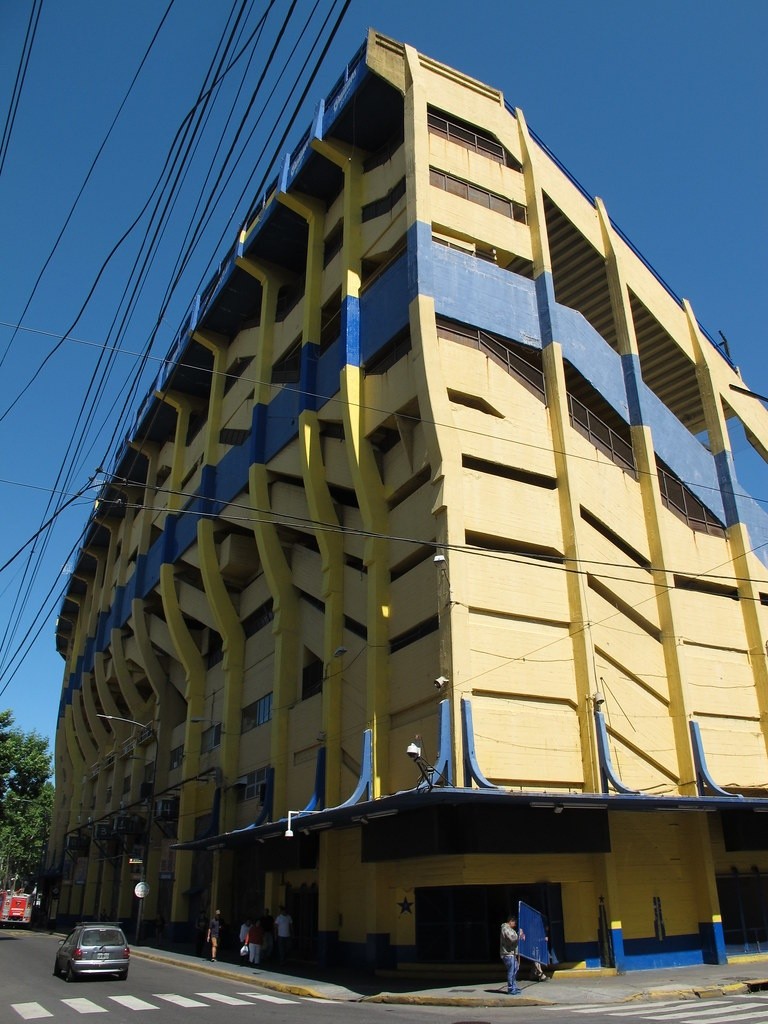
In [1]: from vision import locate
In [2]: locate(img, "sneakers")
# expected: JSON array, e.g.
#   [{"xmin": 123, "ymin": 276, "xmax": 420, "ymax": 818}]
[
  {"xmin": 514, "ymin": 988, "xmax": 521, "ymax": 993},
  {"xmin": 508, "ymin": 990, "xmax": 517, "ymax": 995}
]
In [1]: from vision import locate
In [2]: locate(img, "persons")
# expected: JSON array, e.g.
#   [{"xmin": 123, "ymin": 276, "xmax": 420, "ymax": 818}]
[
  {"xmin": 154, "ymin": 911, "xmax": 165, "ymax": 946},
  {"xmin": 502, "ymin": 916, "xmax": 524, "ymax": 995},
  {"xmin": 101, "ymin": 907, "xmax": 116, "ymax": 921},
  {"xmin": 196, "ymin": 911, "xmax": 209, "ymax": 940},
  {"xmin": 207, "ymin": 910, "xmax": 224, "ymax": 962},
  {"xmin": 240, "ymin": 907, "xmax": 292, "ymax": 965}
]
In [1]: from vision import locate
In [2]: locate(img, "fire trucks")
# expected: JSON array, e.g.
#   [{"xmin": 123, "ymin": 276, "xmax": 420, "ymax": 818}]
[{"xmin": 0, "ymin": 889, "xmax": 34, "ymax": 930}]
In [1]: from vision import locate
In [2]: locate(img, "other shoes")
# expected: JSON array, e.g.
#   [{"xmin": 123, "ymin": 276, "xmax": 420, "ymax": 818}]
[{"xmin": 211, "ymin": 959, "xmax": 218, "ymax": 962}]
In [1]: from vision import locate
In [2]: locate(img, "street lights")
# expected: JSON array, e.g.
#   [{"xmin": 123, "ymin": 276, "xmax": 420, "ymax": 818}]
[
  {"xmin": 13, "ymin": 797, "xmax": 47, "ymax": 880},
  {"xmin": 96, "ymin": 714, "xmax": 159, "ymax": 945}
]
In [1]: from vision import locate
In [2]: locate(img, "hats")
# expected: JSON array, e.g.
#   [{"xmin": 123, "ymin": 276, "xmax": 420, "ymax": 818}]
[{"xmin": 216, "ymin": 909, "xmax": 222, "ymax": 914}]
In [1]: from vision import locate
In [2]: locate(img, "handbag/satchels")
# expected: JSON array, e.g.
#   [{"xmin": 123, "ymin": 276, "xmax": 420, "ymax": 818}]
[{"xmin": 240, "ymin": 945, "xmax": 249, "ymax": 956}]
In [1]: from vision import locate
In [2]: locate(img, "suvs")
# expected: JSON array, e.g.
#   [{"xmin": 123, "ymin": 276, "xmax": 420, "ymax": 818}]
[{"xmin": 54, "ymin": 922, "xmax": 130, "ymax": 981}]
